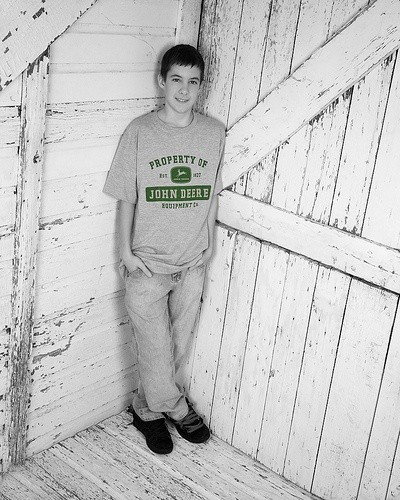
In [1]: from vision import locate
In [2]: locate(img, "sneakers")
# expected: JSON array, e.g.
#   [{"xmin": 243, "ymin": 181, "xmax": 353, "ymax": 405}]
[
  {"xmin": 161, "ymin": 397, "xmax": 210, "ymax": 443},
  {"xmin": 132, "ymin": 409, "xmax": 173, "ymax": 454}
]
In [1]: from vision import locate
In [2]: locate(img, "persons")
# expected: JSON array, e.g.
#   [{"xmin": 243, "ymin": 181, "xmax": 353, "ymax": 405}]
[{"xmin": 103, "ymin": 43, "xmax": 226, "ymax": 455}]
